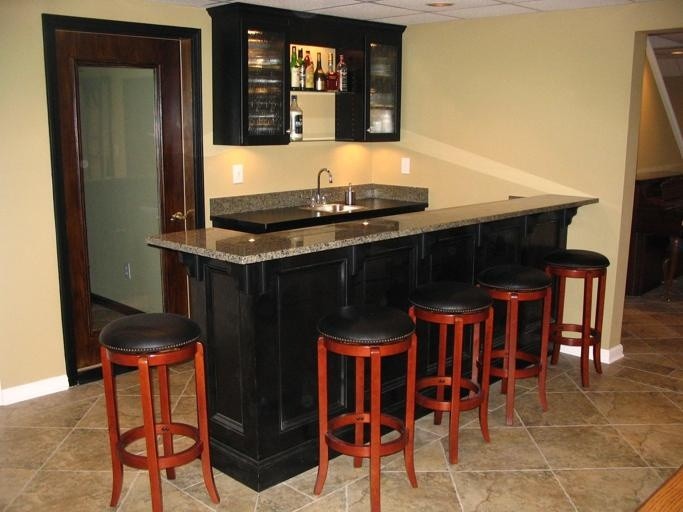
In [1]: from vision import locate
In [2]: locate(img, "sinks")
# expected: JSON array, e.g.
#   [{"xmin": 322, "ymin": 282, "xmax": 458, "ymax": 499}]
[{"xmin": 302, "ymin": 203, "xmax": 364, "ymax": 213}]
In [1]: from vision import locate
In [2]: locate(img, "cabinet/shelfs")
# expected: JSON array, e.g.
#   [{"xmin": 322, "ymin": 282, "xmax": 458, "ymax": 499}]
[
  {"xmin": 206, "ymin": 3, "xmax": 290, "ymax": 145},
  {"xmin": 290, "ymin": 87, "xmax": 354, "ymax": 142},
  {"xmin": 334, "ymin": 17, "xmax": 407, "ymax": 142}
]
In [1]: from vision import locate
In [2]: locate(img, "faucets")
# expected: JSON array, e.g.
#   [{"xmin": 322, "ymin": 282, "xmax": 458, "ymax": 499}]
[{"xmin": 318, "ymin": 169, "xmax": 333, "ymax": 195}]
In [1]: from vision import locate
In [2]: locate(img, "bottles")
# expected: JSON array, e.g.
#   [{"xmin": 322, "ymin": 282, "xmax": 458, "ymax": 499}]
[
  {"xmin": 290, "ymin": 45, "xmax": 348, "ymax": 91},
  {"xmin": 289, "ymin": 96, "xmax": 303, "ymax": 142},
  {"xmin": 344, "ymin": 183, "xmax": 355, "ymax": 205}
]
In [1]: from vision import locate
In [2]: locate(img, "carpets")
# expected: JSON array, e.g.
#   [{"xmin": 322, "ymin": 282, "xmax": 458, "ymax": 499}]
[{"xmin": 635, "ymin": 465, "xmax": 683, "ymax": 512}]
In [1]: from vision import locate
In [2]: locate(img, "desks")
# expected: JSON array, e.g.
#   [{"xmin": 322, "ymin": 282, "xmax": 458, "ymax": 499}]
[{"xmin": 625, "ymin": 168, "xmax": 683, "ymax": 296}]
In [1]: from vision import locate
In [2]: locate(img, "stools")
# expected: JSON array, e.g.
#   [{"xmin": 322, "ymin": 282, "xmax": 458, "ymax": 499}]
[
  {"xmin": 664, "ymin": 234, "xmax": 683, "ymax": 302},
  {"xmin": 469, "ymin": 264, "xmax": 552, "ymax": 426},
  {"xmin": 313, "ymin": 305, "xmax": 418, "ymax": 512},
  {"xmin": 409, "ymin": 280, "xmax": 494, "ymax": 465},
  {"xmin": 544, "ymin": 250, "xmax": 610, "ymax": 387},
  {"xmin": 98, "ymin": 313, "xmax": 220, "ymax": 512}
]
